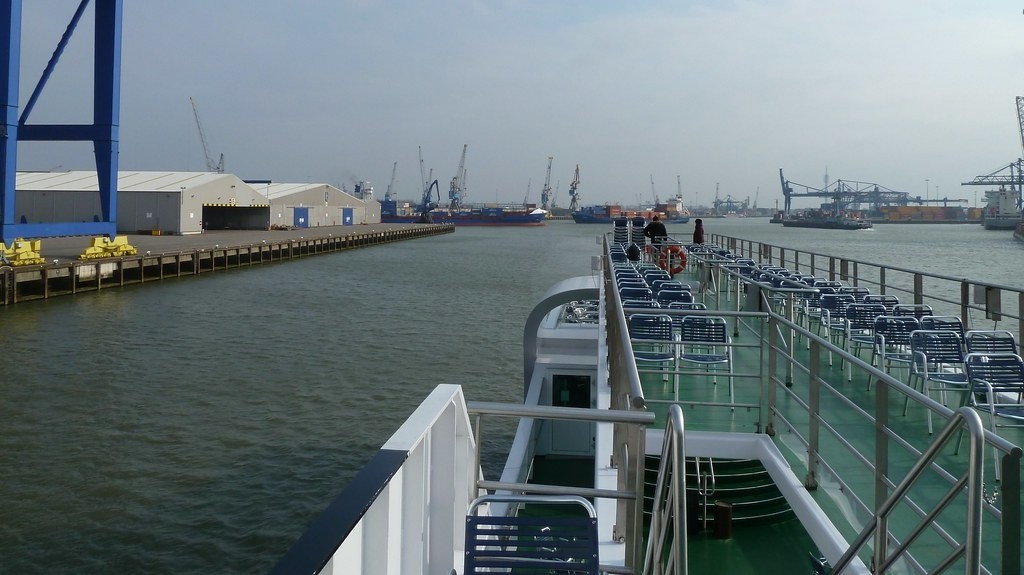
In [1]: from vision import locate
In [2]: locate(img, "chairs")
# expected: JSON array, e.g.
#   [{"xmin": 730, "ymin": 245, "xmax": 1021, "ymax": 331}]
[
  {"xmin": 462, "ymin": 493, "xmax": 600, "ymax": 575},
  {"xmin": 608, "ymin": 216, "xmax": 1023, "ymax": 484}
]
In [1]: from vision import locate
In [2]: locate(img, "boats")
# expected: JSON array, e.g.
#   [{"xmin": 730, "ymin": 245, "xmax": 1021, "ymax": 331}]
[
  {"xmin": 768, "ymin": 214, "xmax": 783, "ymax": 223},
  {"xmin": 783, "ymin": 208, "xmax": 873, "ymax": 230},
  {"xmin": 979, "ymin": 185, "xmax": 1023, "ymax": 230},
  {"xmin": 572, "ymin": 195, "xmax": 692, "ymax": 226},
  {"xmin": 381, "ymin": 202, "xmax": 549, "ymax": 229}
]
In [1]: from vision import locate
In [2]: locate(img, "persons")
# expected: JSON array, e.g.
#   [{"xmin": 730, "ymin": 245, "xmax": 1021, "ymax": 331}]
[
  {"xmin": 693, "ymin": 219, "xmax": 704, "ymax": 266},
  {"xmin": 644, "ymin": 215, "xmax": 667, "ymax": 263}
]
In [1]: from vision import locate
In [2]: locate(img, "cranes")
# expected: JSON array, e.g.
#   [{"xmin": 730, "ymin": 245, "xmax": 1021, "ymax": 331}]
[
  {"xmin": 537, "ymin": 155, "xmax": 563, "ymax": 219},
  {"xmin": 714, "ymin": 182, "xmax": 751, "ymax": 217},
  {"xmin": 773, "ymin": 169, "xmax": 970, "ymax": 216},
  {"xmin": 569, "ymin": 165, "xmax": 583, "ymax": 214},
  {"xmin": 377, "ymin": 143, "xmax": 474, "ymax": 217},
  {"xmin": 958, "ymin": 96, "xmax": 1024, "ymax": 212},
  {"xmin": 189, "ymin": 96, "xmax": 225, "ymax": 174}
]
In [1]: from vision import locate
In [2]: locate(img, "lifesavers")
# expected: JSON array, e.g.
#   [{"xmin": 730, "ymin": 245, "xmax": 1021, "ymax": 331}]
[{"xmin": 659, "ymin": 248, "xmax": 686, "ymax": 273}]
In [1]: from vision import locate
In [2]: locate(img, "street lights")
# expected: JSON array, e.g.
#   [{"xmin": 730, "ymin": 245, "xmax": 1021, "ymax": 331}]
[{"xmin": 926, "ymin": 179, "xmax": 930, "ymax": 207}]
